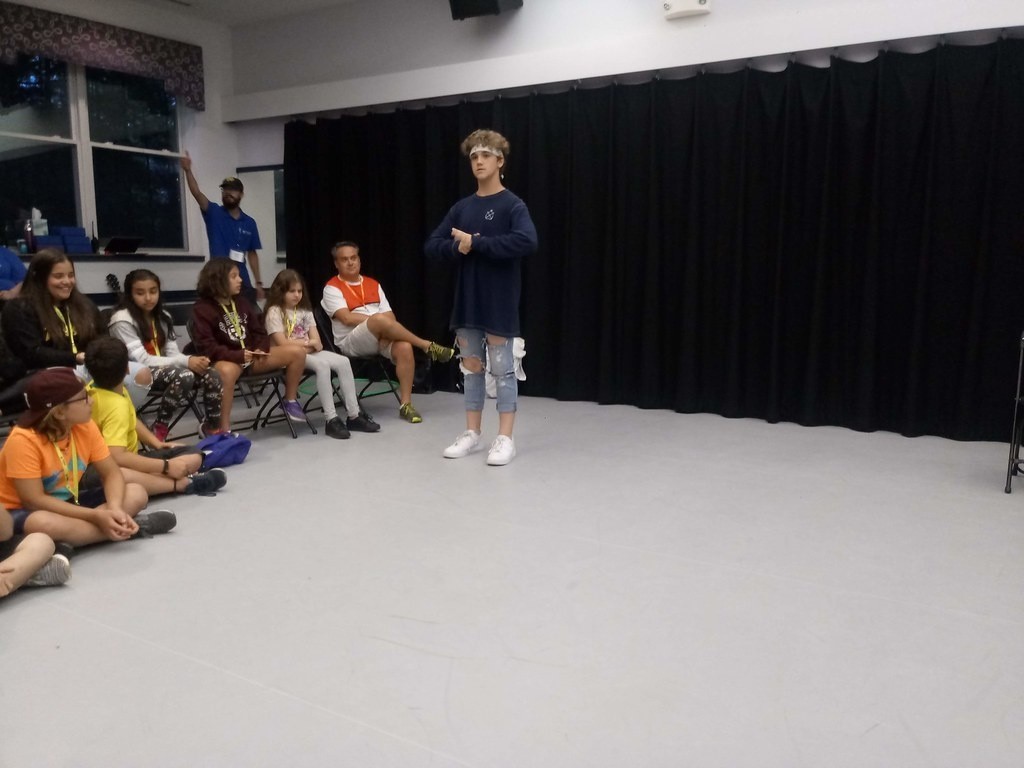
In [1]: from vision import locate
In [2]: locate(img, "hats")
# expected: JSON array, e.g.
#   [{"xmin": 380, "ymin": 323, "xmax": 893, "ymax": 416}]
[
  {"xmin": 16, "ymin": 366, "xmax": 86, "ymax": 428},
  {"xmin": 219, "ymin": 178, "xmax": 244, "ymax": 192}
]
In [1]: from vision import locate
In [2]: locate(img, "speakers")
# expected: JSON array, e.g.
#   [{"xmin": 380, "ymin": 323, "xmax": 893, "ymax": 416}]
[{"xmin": 449, "ymin": 0, "xmax": 523, "ymax": 21}]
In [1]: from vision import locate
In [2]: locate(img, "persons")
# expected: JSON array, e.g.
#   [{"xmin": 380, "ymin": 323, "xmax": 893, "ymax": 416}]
[
  {"xmin": 107, "ymin": 270, "xmax": 224, "ymax": 442},
  {"xmin": 0, "ymin": 241, "xmax": 28, "ymax": 300},
  {"xmin": 320, "ymin": 242, "xmax": 456, "ymax": 423},
  {"xmin": 1, "ymin": 503, "xmax": 70, "ymax": 599},
  {"xmin": 188, "ymin": 255, "xmax": 306, "ymax": 433},
  {"xmin": 22, "ymin": 248, "xmax": 112, "ymax": 367},
  {"xmin": 422, "ymin": 130, "xmax": 537, "ymax": 466},
  {"xmin": 179, "ymin": 149, "xmax": 265, "ymax": 302},
  {"xmin": 81, "ymin": 336, "xmax": 227, "ymax": 497},
  {"xmin": 0, "ymin": 367, "xmax": 176, "ymax": 546},
  {"xmin": 263, "ymin": 268, "xmax": 380, "ymax": 439}
]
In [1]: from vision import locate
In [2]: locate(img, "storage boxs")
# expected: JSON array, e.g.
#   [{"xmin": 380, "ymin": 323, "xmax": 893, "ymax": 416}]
[
  {"xmin": 36, "ymin": 245, "xmax": 65, "ymax": 253},
  {"xmin": 51, "ymin": 227, "xmax": 86, "ymax": 237},
  {"xmin": 63, "ymin": 237, "xmax": 90, "ymax": 245},
  {"xmin": 33, "ymin": 236, "xmax": 63, "ymax": 245},
  {"xmin": 66, "ymin": 245, "xmax": 92, "ymax": 253}
]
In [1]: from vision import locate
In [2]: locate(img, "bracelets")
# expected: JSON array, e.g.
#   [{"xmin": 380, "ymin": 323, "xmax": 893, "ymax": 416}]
[
  {"xmin": 163, "ymin": 457, "xmax": 168, "ymax": 475},
  {"xmin": 256, "ymin": 281, "xmax": 262, "ymax": 285}
]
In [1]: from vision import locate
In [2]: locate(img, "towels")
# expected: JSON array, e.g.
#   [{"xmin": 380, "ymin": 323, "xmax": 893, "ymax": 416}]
[{"xmin": 484, "ymin": 336, "xmax": 527, "ymax": 399}]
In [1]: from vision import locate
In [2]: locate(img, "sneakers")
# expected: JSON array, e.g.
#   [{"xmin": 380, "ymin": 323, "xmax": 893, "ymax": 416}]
[
  {"xmin": 346, "ymin": 411, "xmax": 380, "ymax": 432},
  {"xmin": 487, "ymin": 434, "xmax": 515, "ymax": 465},
  {"xmin": 443, "ymin": 430, "xmax": 484, "ymax": 459},
  {"xmin": 425, "ymin": 343, "xmax": 455, "ymax": 362},
  {"xmin": 129, "ymin": 509, "xmax": 177, "ymax": 539},
  {"xmin": 55, "ymin": 543, "xmax": 75, "ymax": 560},
  {"xmin": 151, "ymin": 421, "xmax": 168, "ymax": 442},
  {"xmin": 399, "ymin": 402, "xmax": 422, "ymax": 423},
  {"xmin": 280, "ymin": 400, "xmax": 307, "ymax": 421},
  {"xmin": 201, "ymin": 423, "xmax": 220, "ymax": 437},
  {"xmin": 325, "ymin": 416, "xmax": 351, "ymax": 439},
  {"xmin": 185, "ymin": 467, "xmax": 228, "ymax": 497},
  {"xmin": 19, "ymin": 555, "xmax": 71, "ymax": 587}
]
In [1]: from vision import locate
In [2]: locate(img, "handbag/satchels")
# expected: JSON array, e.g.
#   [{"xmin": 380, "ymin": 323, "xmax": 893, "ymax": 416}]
[{"xmin": 195, "ymin": 430, "xmax": 251, "ymax": 472}]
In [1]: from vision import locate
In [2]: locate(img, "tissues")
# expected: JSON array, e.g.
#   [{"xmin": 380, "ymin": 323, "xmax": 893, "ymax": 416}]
[{"xmin": 30, "ymin": 207, "xmax": 49, "ymax": 237}]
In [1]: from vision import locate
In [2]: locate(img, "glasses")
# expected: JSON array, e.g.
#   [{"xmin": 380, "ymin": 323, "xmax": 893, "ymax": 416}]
[{"xmin": 64, "ymin": 390, "xmax": 91, "ymax": 406}]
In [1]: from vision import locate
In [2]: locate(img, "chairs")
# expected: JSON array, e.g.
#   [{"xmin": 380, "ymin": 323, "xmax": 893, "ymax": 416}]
[{"xmin": 80, "ymin": 287, "xmax": 401, "ymax": 453}]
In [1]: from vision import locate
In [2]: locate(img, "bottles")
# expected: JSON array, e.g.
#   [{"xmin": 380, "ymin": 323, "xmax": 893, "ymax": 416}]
[
  {"xmin": 19, "ymin": 239, "xmax": 27, "ymax": 254},
  {"xmin": 24, "ymin": 219, "xmax": 33, "ymax": 250}
]
